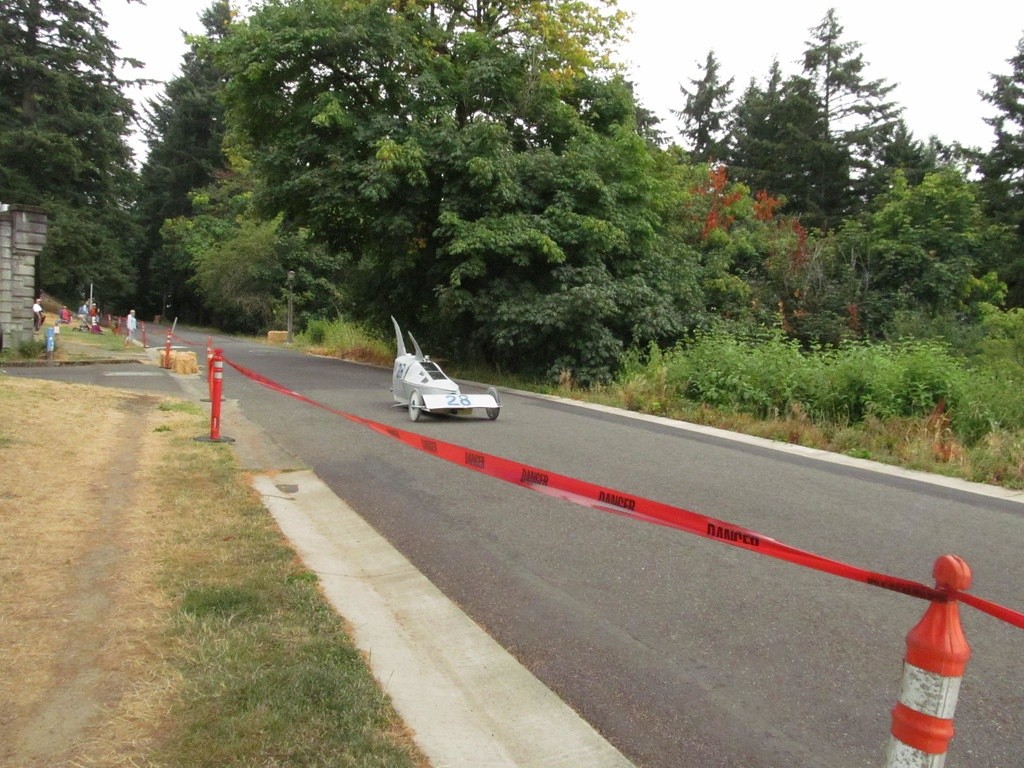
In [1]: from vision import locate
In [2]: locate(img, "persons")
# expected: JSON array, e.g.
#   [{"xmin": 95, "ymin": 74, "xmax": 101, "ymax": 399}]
[
  {"xmin": 123, "ymin": 309, "xmax": 137, "ymax": 346},
  {"xmin": 77, "ymin": 300, "xmax": 105, "ymax": 335},
  {"xmin": 59, "ymin": 305, "xmax": 73, "ymax": 324},
  {"xmin": 33, "ymin": 298, "xmax": 43, "ymax": 335}
]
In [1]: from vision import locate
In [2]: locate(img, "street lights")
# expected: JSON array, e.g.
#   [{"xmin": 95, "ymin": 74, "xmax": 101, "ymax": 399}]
[{"xmin": 285, "ymin": 271, "xmax": 297, "ymax": 347}]
